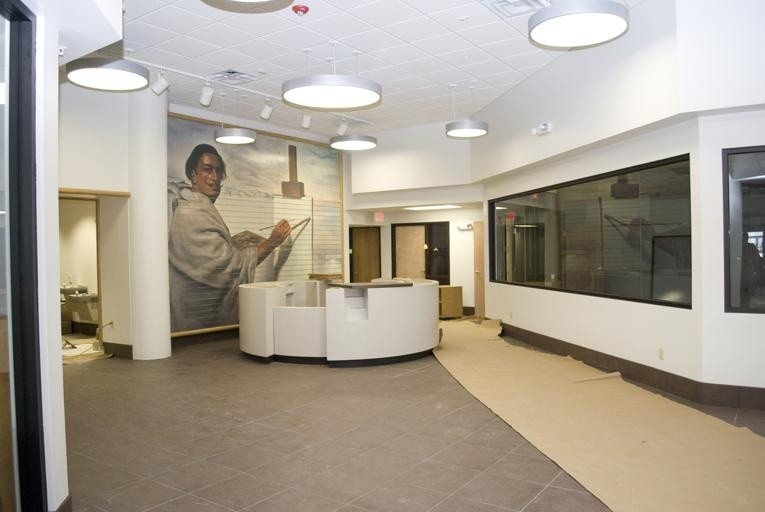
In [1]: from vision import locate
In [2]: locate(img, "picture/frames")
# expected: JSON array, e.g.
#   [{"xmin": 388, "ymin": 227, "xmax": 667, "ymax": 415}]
[{"xmin": 168, "ymin": 111, "xmax": 345, "ymax": 339}]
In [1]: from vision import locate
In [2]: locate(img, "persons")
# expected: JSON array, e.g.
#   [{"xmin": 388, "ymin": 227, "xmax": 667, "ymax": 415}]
[{"xmin": 168, "ymin": 143, "xmax": 291, "ymax": 333}]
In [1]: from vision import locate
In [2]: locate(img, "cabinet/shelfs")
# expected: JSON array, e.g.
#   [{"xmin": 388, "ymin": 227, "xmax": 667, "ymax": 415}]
[{"xmin": 438, "ymin": 284, "xmax": 463, "ymax": 320}]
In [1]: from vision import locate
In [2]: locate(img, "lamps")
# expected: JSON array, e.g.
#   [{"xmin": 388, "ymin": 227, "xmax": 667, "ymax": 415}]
[
  {"xmin": 259, "ymin": 98, "xmax": 273, "ymax": 121},
  {"xmin": 65, "ymin": 46, "xmax": 149, "ymax": 92},
  {"xmin": 446, "ymin": 85, "xmax": 488, "ymax": 140},
  {"xmin": 337, "ymin": 118, "xmax": 347, "ymax": 136},
  {"xmin": 301, "ymin": 112, "xmax": 312, "ymax": 129},
  {"xmin": 526, "ymin": 1, "xmax": 630, "ymax": 52},
  {"xmin": 330, "ymin": 112, "xmax": 378, "ymax": 152},
  {"xmin": 199, "ymin": 81, "xmax": 214, "ymax": 107},
  {"xmin": 150, "ymin": 69, "xmax": 170, "ymax": 96},
  {"xmin": 281, "ymin": 42, "xmax": 381, "ymax": 110},
  {"xmin": 215, "ymin": 93, "xmax": 256, "ymax": 145}
]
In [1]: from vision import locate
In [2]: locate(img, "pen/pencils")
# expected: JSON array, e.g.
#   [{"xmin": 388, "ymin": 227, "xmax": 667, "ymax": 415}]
[{"xmin": 260, "ymin": 219, "xmax": 296, "ymax": 231}]
